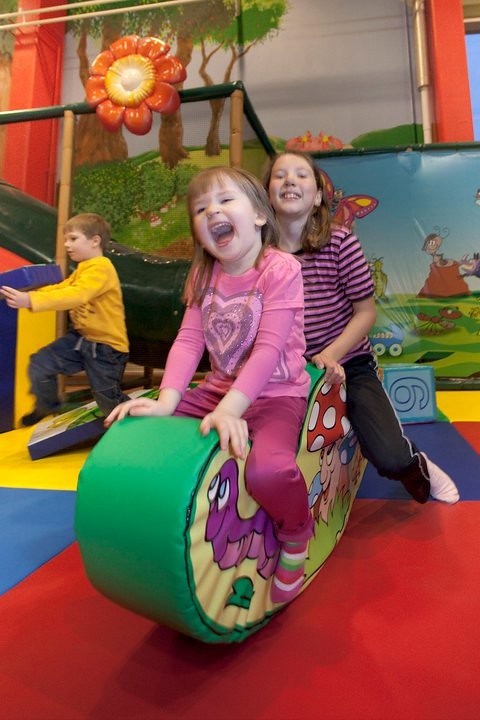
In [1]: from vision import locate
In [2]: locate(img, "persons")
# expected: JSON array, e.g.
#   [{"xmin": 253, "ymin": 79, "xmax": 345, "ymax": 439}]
[
  {"xmin": 104, "ymin": 165, "xmax": 312, "ymax": 602},
  {"xmin": 0, "ymin": 213, "xmax": 133, "ymax": 426},
  {"xmin": 261, "ymin": 148, "xmax": 459, "ymax": 504}
]
